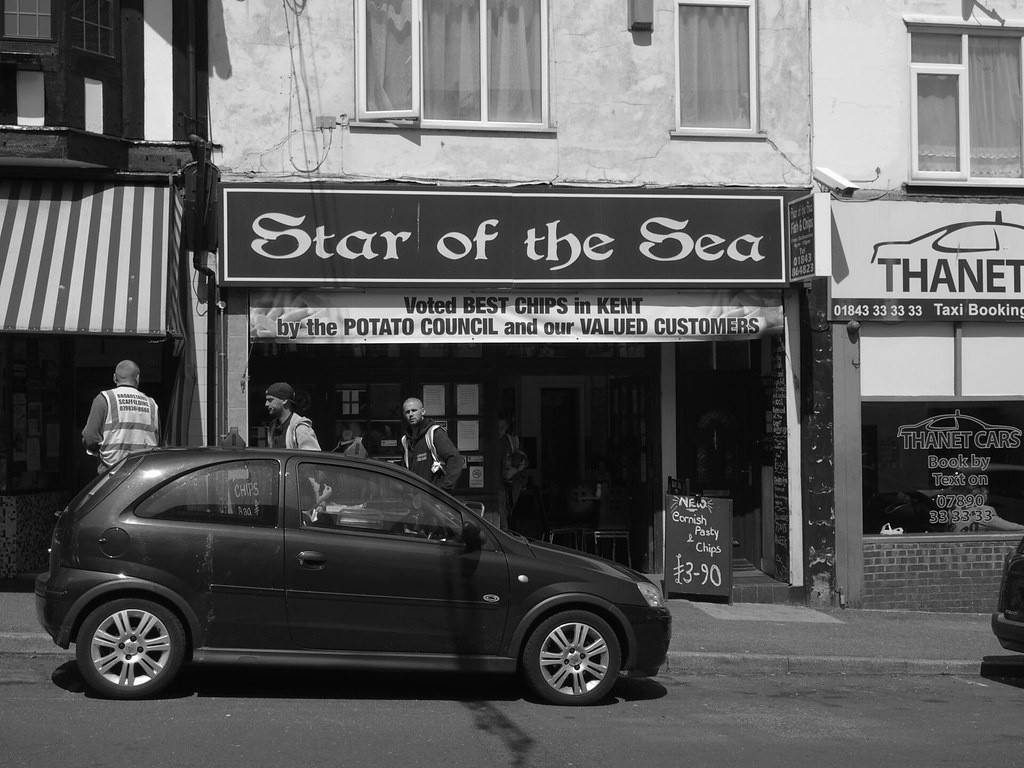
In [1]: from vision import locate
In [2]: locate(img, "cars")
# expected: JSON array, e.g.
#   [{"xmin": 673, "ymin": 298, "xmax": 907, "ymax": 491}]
[
  {"xmin": 917, "ymin": 463, "xmax": 1024, "ymax": 509},
  {"xmin": 991, "ymin": 534, "xmax": 1024, "ymax": 650},
  {"xmin": 35, "ymin": 445, "xmax": 673, "ymax": 706}
]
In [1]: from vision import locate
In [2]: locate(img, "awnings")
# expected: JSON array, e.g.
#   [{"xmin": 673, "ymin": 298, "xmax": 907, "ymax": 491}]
[{"xmin": 0, "ymin": 173, "xmax": 187, "ymax": 354}]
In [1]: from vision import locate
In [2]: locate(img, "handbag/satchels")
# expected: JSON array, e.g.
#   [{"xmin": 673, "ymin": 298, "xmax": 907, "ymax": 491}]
[{"xmin": 291, "ymin": 422, "xmax": 332, "ymax": 511}]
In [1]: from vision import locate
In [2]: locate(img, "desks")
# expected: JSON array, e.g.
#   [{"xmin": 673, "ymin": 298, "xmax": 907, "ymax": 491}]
[{"xmin": 559, "ymin": 494, "xmax": 630, "ymax": 556}]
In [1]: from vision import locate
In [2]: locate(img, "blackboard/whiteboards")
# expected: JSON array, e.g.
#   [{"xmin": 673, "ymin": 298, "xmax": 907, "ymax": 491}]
[{"xmin": 662, "ymin": 494, "xmax": 734, "ymax": 598}]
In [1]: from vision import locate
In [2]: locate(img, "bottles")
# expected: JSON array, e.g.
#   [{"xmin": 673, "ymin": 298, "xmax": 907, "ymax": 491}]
[{"xmin": 596, "ymin": 483, "xmax": 601, "ymax": 496}]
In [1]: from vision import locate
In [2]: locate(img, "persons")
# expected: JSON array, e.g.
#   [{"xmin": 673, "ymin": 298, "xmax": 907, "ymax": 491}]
[
  {"xmin": 946, "ymin": 482, "xmax": 1024, "ymax": 531},
  {"xmin": 265, "ymin": 383, "xmax": 332, "ymax": 513},
  {"xmin": 498, "ymin": 413, "xmax": 536, "ymax": 532},
  {"xmin": 81, "ymin": 361, "xmax": 162, "ymax": 476},
  {"xmin": 399, "ymin": 398, "xmax": 461, "ymax": 497}
]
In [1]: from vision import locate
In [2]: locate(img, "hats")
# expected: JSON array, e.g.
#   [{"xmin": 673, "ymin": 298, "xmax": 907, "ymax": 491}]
[{"xmin": 264, "ymin": 382, "xmax": 298, "ymax": 406}]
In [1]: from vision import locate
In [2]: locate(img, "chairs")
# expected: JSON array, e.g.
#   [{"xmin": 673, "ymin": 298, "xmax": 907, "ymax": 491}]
[
  {"xmin": 261, "ymin": 476, "xmax": 317, "ymax": 528},
  {"xmin": 532, "ymin": 486, "xmax": 632, "ymax": 570}
]
